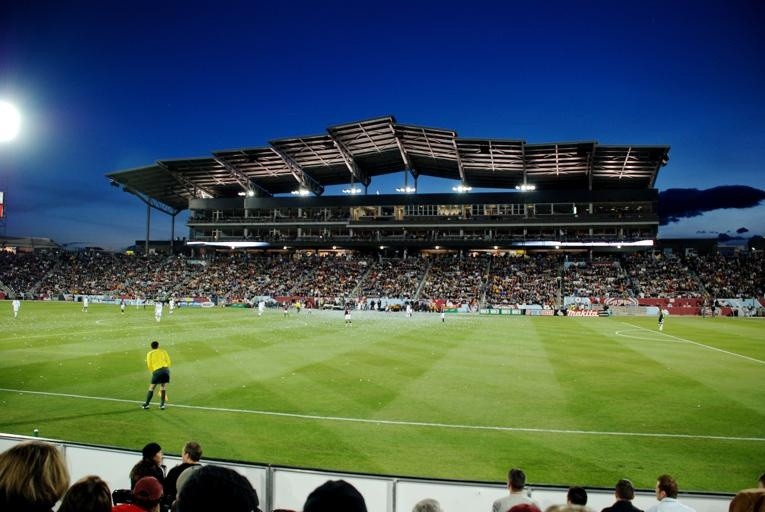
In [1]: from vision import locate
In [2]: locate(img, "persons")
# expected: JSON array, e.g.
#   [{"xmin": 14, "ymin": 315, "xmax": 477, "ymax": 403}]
[
  {"xmin": 729, "ymin": 472, "xmax": 764, "ymax": 512},
  {"xmin": 492, "ymin": 468, "xmax": 596, "ymax": 512},
  {"xmin": 601, "ymin": 479, "xmax": 645, "ymax": 512},
  {"xmin": 1, "ymin": 441, "xmax": 368, "ymax": 512},
  {"xmin": 412, "ymin": 498, "xmax": 444, "ymax": 512},
  {"xmin": 645, "ymin": 474, "xmax": 697, "ymax": 512},
  {"xmin": 141, "ymin": 340, "xmax": 172, "ymax": 410},
  {"xmin": 1, "ymin": 249, "xmax": 765, "ymax": 331}
]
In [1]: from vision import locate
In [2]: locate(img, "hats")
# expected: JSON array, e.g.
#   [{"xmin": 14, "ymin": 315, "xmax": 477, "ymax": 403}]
[{"xmin": 134, "ymin": 476, "xmax": 162, "ymax": 500}]
